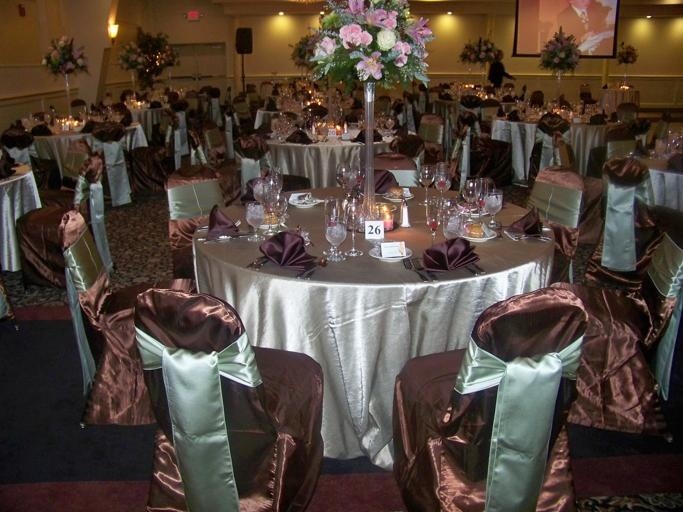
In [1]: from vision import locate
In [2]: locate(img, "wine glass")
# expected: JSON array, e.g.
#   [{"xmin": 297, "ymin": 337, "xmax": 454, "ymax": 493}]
[
  {"xmin": 419, "ymin": 163, "xmax": 503, "ymax": 242},
  {"xmin": 245, "ymin": 167, "xmax": 288, "ymax": 242},
  {"xmin": 655, "ymin": 139, "xmax": 666, "ymax": 162},
  {"xmin": 323, "ymin": 163, "xmax": 364, "ymax": 262}
]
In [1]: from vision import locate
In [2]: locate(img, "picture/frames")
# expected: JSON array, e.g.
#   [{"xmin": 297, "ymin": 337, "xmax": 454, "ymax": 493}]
[{"xmin": 512, "ymin": 0, "xmax": 620, "ymax": 59}]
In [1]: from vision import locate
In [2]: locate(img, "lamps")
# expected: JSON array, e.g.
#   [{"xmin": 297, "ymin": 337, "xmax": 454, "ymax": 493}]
[{"xmin": 109, "ymin": 23, "xmax": 119, "ymax": 47}]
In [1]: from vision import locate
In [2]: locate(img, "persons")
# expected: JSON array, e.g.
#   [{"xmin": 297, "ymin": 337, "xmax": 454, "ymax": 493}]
[{"xmin": 557, "ymin": 0, "xmax": 606, "ymax": 55}]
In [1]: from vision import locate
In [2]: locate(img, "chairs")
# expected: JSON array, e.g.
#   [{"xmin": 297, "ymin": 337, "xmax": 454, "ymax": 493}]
[
  {"xmin": 551, "ymin": 230, "xmax": 683, "ymax": 450},
  {"xmin": 132, "ymin": 288, "xmax": 326, "ymax": 511},
  {"xmin": 390, "ymin": 288, "xmax": 592, "ymax": 509},
  {"xmin": 15, "ymin": 155, "xmax": 114, "ymax": 295},
  {"xmin": 166, "ymin": 176, "xmax": 226, "ymax": 291},
  {"xmin": 8, "ymin": 91, "xmax": 683, "ymax": 199},
  {"xmin": 524, "ymin": 166, "xmax": 584, "ymax": 285},
  {"xmin": 585, "ymin": 157, "xmax": 678, "ymax": 289},
  {"xmin": 58, "ymin": 211, "xmax": 196, "ymax": 429}
]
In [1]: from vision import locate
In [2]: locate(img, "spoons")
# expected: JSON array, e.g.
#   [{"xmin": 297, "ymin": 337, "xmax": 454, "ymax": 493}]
[{"xmin": 305, "ymin": 257, "xmax": 328, "ymax": 281}]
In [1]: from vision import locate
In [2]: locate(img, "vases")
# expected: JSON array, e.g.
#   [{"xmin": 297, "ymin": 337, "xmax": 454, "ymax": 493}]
[
  {"xmin": 64, "ymin": 74, "xmax": 73, "ymax": 118},
  {"xmin": 554, "ymin": 69, "xmax": 563, "ymax": 107},
  {"xmin": 478, "ymin": 61, "xmax": 487, "ymax": 94},
  {"xmin": 358, "ymin": 80, "xmax": 380, "ymax": 235},
  {"xmin": 623, "ymin": 63, "xmax": 629, "ymax": 85}
]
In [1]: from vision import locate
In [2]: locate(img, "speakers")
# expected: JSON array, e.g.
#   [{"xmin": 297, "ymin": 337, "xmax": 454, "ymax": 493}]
[{"xmin": 236, "ymin": 27, "xmax": 253, "ymax": 54}]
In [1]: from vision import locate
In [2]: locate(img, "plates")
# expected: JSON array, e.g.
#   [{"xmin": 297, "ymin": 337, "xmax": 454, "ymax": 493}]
[
  {"xmin": 289, "ymin": 198, "xmax": 324, "ymax": 209},
  {"xmin": 382, "ymin": 194, "xmax": 414, "ymax": 202},
  {"xmin": 369, "ymin": 246, "xmax": 413, "ymax": 261}
]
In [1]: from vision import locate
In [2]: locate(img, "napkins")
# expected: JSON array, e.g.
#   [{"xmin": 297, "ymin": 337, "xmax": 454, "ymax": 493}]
[
  {"xmin": 241, "ymin": 177, "xmax": 266, "ymax": 205},
  {"xmin": 205, "ymin": 206, "xmax": 240, "ymax": 240},
  {"xmin": 421, "ymin": 237, "xmax": 480, "ymax": 270},
  {"xmin": 511, "ymin": 207, "xmax": 544, "ymax": 237},
  {"xmin": 259, "ymin": 233, "xmax": 314, "ymax": 272}
]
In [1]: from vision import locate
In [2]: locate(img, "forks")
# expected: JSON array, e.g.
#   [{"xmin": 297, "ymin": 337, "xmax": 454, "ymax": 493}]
[
  {"xmin": 403, "ymin": 259, "xmax": 438, "ymax": 282},
  {"xmin": 197, "ymin": 220, "xmax": 241, "ymax": 230},
  {"xmin": 504, "ymin": 231, "xmax": 552, "ymax": 245}
]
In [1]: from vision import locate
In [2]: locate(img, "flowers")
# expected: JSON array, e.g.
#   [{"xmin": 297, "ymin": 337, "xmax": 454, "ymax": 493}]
[
  {"xmin": 537, "ymin": 22, "xmax": 581, "ymax": 79},
  {"xmin": 287, "ymin": 0, "xmax": 429, "ymax": 92},
  {"xmin": 108, "ymin": 27, "xmax": 181, "ymax": 85},
  {"xmin": 458, "ymin": 35, "xmax": 505, "ymax": 72},
  {"xmin": 616, "ymin": 44, "xmax": 638, "ymax": 67},
  {"xmin": 37, "ymin": 34, "xmax": 90, "ymax": 81}
]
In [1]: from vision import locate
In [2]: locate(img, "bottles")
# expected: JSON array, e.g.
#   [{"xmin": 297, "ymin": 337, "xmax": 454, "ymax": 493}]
[{"xmin": 399, "ymin": 199, "xmax": 410, "ymax": 227}]
